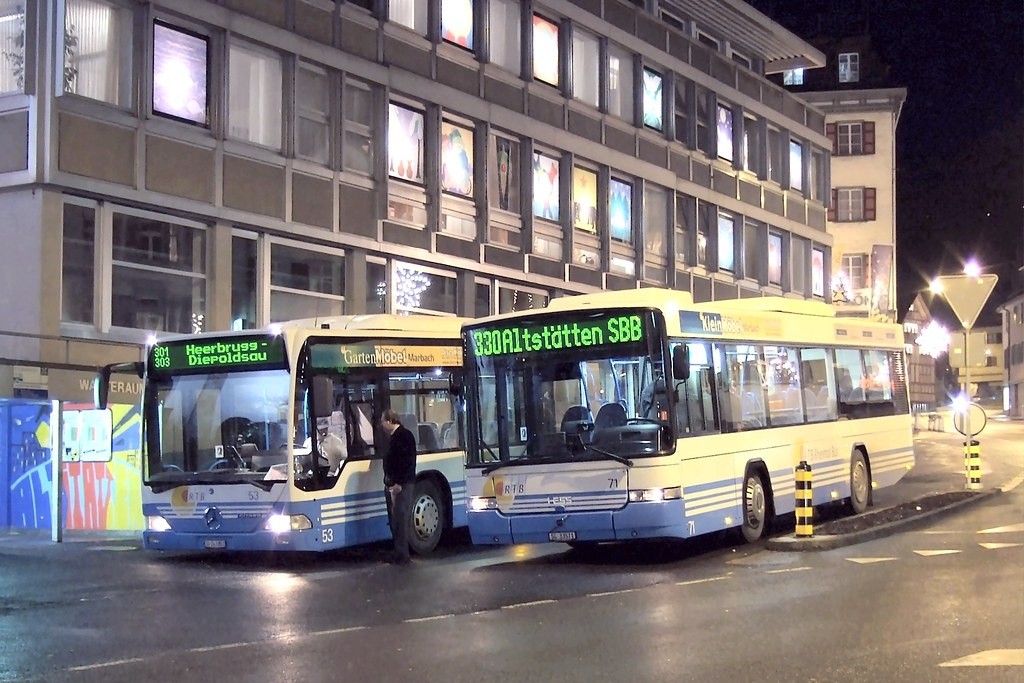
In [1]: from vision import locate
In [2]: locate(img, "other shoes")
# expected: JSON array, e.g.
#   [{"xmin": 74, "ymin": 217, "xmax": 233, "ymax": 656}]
[{"xmin": 378, "ymin": 552, "xmax": 410, "ymax": 564}]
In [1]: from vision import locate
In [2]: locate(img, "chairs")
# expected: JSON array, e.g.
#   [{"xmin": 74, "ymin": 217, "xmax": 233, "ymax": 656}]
[
  {"xmin": 440, "ymin": 422, "xmax": 453, "ymax": 445},
  {"xmin": 593, "ymin": 403, "xmax": 627, "ymax": 440},
  {"xmin": 741, "ymin": 386, "xmax": 862, "ymax": 417},
  {"xmin": 418, "ymin": 423, "xmax": 442, "ymax": 448},
  {"xmin": 560, "ymin": 406, "xmax": 593, "ymax": 443}
]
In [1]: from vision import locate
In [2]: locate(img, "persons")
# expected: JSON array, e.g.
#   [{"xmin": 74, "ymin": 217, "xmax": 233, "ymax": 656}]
[
  {"xmin": 378, "ymin": 409, "xmax": 416, "ymax": 564},
  {"xmin": 638, "ymin": 365, "xmax": 669, "ymax": 425},
  {"xmin": 296, "ymin": 417, "xmax": 348, "ymax": 477}
]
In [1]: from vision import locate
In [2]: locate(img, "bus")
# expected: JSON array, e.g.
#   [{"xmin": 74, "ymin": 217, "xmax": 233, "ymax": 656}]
[
  {"xmin": 94, "ymin": 308, "xmax": 485, "ymax": 553},
  {"xmin": 449, "ymin": 288, "xmax": 916, "ymax": 560}
]
